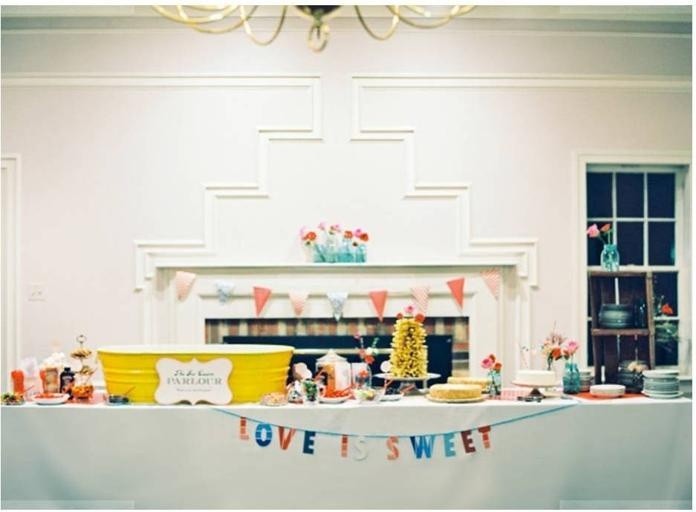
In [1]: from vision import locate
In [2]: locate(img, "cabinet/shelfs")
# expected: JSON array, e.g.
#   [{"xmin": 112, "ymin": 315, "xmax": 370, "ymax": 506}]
[{"xmin": 585, "ymin": 162, "xmax": 691, "ymax": 389}]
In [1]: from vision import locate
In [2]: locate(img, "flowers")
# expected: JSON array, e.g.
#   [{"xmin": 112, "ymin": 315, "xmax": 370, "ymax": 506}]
[
  {"xmin": 355, "ymin": 334, "xmax": 379, "ymax": 382},
  {"xmin": 480, "ymin": 355, "xmax": 501, "ymax": 387},
  {"xmin": 301, "ymin": 217, "xmax": 370, "ymax": 250},
  {"xmin": 587, "ymin": 221, "xmax": 619, "ymax": 269},
  {"xmin": 539, "ymin": 334, "xmax": 579, "ymax": 381}
]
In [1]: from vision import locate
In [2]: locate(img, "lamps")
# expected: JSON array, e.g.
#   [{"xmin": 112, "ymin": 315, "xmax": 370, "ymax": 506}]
[{"xmin": 146, "ymin": 5, "xmax": 478, "ymax": 54}]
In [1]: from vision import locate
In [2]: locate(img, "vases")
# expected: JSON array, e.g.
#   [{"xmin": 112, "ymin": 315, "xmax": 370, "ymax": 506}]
[
  {"xmin": 562, "ymin": 362, "xmax": 581, "ymax": 394},
  {"xmin": 486, "ymin": 370, "xmax": 503, "ymax": 397},
  {"xmin": 354, "ymin": 362, "xmax": 372, "ymax": 391},
  {"xmin": 309, "ymin": 244, "xmax": 365, "ymax": 265},
  {"xmin": 600, "ymin": 246, "xmax": 620, "ymax": 271}
]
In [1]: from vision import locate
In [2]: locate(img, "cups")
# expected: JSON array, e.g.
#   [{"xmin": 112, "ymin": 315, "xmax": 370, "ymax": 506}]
[{"xmin": 300, "ymin": 380, "xmax": 326, "ymax": 406}]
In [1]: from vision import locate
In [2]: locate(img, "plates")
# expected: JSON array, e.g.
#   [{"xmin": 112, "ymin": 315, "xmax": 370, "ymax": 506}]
[
  {"xmin": 380, "ymin": 388, "xmax": 405, "ymax": 401},
  {"xmin": 425, "ymin": 393, "xmax": 489, "ymax": 402},
  {"xmin": 590, "ymin": 384, "xmax": 626, "ymax": 398},
  {"xmin": 641, "ymin": 390, "xmax": 684, "ymax": 398},
  {"xmin": 32, "ymin": 392, "xmax": 70, "ymax": 405},
  {"xmin": 318, "ymin": 391, "xmax": 352, "ymax": 404}
]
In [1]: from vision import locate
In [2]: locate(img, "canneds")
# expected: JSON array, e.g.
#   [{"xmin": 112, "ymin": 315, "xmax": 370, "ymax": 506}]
[{"xmin": 44, "ymin": 367, "xmax": 58, "ymax": 393}]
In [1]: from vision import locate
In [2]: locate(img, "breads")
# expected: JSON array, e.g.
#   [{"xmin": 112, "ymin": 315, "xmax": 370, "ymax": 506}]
[
  {"xmin": 429, "ymin": 383, "xmax": 481, "ymax": 399},
  {"xmin": 448, "ymin": 377, "xmax": 491, "ymax": 389}
]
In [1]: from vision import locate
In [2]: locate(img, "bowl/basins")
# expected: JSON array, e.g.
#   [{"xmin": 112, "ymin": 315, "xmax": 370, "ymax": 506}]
[
  {"xmin": 1, "ymin": 392, "xmax": 26, "ymax": 406},
  {"xmin": 350, "ymin": 385, "xmax": 386, "ymax": 404},
  {"xmin": 104, "ymin": 393, "xmax": 134, "ymax": 406}
]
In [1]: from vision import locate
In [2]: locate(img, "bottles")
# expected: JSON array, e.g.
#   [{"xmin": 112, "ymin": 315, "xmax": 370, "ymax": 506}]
[
  {"xmin": 73, "ymin": 370, "xmax": 95, "ymax": 400},
  {"xmin": 39, "ymin": 366, "xmax": 75, "ymax": 400},
  {"xmin": 10, "ymin": 369, "xmax": 25, "ymax": 394}
]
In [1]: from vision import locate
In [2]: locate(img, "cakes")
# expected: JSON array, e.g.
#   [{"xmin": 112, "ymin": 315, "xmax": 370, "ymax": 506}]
[{"xmin": 514, "ymin": 370, "xmax": 555, "ymax": 384}]
